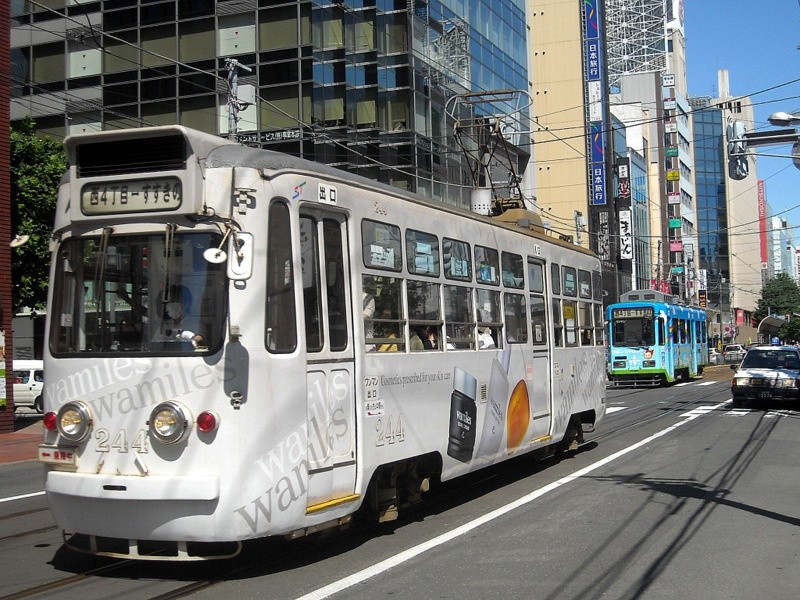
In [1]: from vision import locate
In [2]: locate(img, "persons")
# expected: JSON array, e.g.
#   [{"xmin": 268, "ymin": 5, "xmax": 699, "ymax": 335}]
[
  {"xmin": 150, "ymin": 287, "xmax": 208, "ymax": 347},
  {"xmin": 360, "ymin": 286, "xmax": 515, "ymax": 353},
  {"xmin": 740, "ymin": 349, "xmax": 747, "ymax": 361}
]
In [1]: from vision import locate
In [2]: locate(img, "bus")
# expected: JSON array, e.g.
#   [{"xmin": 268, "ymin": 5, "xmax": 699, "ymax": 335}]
[
  {"xmin": 38, "ymin": 118, "xmax": 607, "ymax": 560},
  {"xmin": 605, "ymin": 299, "xmax": 709, "ymax": 389}
]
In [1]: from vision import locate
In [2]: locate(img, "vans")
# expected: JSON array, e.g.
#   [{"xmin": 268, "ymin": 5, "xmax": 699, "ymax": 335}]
[{"xmin": 11, "ymin": 360, "xmax": 45, "ymax": 413}]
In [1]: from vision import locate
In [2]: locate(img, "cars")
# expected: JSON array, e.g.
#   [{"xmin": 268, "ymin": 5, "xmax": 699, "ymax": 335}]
[
  {"xmin": 709, "ymin": 343, "xmax": 748, "ymax": 365},
  {"xmin": 730, "ymin": 345, "xmax": 800, "ymax": 408}
]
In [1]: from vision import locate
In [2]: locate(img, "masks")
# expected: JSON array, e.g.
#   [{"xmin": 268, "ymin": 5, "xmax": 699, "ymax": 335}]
[{"xmin": 166, "ymin": 302, "xmax": 185, "ymax": 319}]
